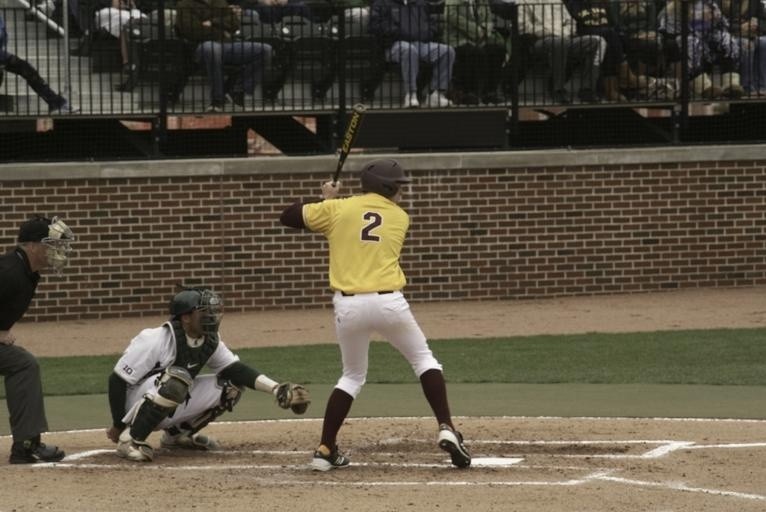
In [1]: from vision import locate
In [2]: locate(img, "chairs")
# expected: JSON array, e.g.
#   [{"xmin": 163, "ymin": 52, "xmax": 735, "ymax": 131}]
[{"xmin": 130, "ymin": 16, "xmax": 386, "ymax": 102}]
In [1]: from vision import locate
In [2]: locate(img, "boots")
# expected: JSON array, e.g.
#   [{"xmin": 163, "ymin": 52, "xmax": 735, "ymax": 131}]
[{"xmin": 552, "ymin": 63, "xmax": 764, "ymax": 105}]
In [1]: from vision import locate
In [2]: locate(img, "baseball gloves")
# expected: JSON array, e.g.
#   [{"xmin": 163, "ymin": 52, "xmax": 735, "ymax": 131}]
[{"xmin": 276, "ymin": 381, "xmax": 311, "ymax": 414}]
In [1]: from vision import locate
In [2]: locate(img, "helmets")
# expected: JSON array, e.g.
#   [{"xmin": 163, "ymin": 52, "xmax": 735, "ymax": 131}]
[
  {"xmin": 18, "ymin": 215, "xmax": 75, "ymax": 277},
  {"xmin": 169, "ymin": 288, "xmax": 224, "ymax": 346},
  {"xmin": 361, "ymin": 159, "xmax": 413, "ymax": 198}
]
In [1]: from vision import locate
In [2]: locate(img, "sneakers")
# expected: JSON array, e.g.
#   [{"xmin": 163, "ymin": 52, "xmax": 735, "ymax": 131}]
[
  {"xmin": 311, "ymin": 446, "xmax": 350, "ymax": 472},
  {"xmin": 114, "ymin": 439, "xmax": 148, "ymax": 462},
  {"xmin": 403, "ymin": 87, "xmax": 504, "ymax": 107},
  {"xmin": 437, "ymin": 423, "xmax": 471, "ymax": 468},
  {"xmin": 204, "ymin": 90, "xmax": 244, "ymax": 114},
  {"xmin": 161, "ymin": 433, "xmax": 215, "ymax": 449},
  {"xmin": 9, "ymin": 441, "xmax": 65, "ymax": 463}
]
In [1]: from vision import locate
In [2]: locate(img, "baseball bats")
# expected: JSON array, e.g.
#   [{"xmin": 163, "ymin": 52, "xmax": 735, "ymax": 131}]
[{"xmin": 332, "ymin": 103, "xmax": 365, "ymax": 186}]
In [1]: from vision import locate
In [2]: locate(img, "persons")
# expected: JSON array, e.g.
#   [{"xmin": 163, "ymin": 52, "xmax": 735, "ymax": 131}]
[
  {"xmin": 27, "ymin": 1, "xmax": 766, "ymax": 106},
  {"xmin": 279, "ymin": 157, "xmax": 473, "ymax": 472},
  {"xmin": 104, "ymin": 288, "xmax": 313, "ymax": 462},
  {"xmin": 0, "ymin": 213, "xmax": 76, "ymax": 465}
]
